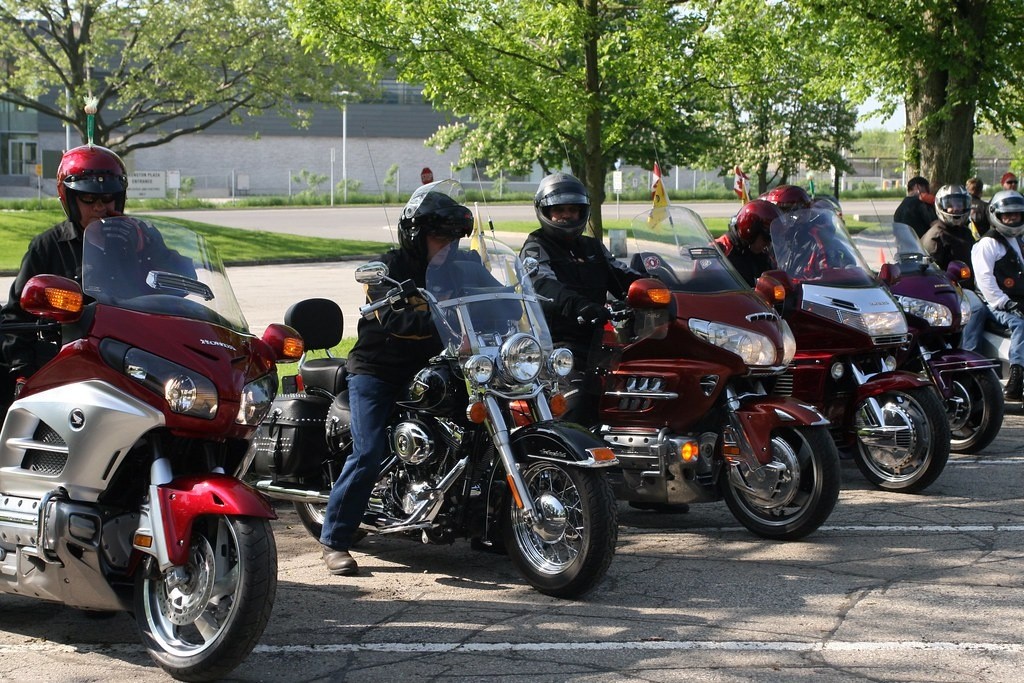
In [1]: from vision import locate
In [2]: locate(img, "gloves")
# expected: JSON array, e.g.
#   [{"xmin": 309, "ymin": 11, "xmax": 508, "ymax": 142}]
[
  {"xmin": 574, "ymin": 301, "xmax": 613, "ymax": 325},
  {"xmin": 1005, "ymin": 300, "xmax": 1024, "ymax": 318}
]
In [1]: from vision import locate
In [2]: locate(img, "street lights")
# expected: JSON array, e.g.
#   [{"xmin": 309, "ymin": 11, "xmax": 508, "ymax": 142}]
[{"xmin": 339, "ymin": 90, "xmax": 349, "ymax": 203}]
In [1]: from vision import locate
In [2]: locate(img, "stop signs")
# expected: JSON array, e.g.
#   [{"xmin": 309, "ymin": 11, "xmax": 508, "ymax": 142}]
[{"xmin": 421, "ymin": 168, "xmax": 433, "ymax": 185}]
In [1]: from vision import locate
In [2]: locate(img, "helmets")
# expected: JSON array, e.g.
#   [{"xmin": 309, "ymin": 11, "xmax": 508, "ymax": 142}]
[
  {"xmin": 808, "ymin": 195, "xmax": 842, "ymax": 241},
  {"xmin": 987, "ymin": 191, "xmax": 1024, "ymax": 237},
  {"xmin": 768, "ymin": 185, "xmax": 810, "ymax": 212},
  {"xmin": 756, "ymin": 191, "xmax": 769, "ymax": 201},
  {"xmin": 398, "ymin": 178, "xmax": 474, "ymax": 259},
  {"xmin": 57, "ymin": 144, "xmax": 128, "ymax": 222},
  {"xmin": 935, "ymin": 184, "xmax": 972, "ymax": 227},
  {"xmin": 737, "ymin": 200, "xmax": 783, "ymax": 252},
  {"xmin": 535, "ymin": 173, "xmax": 591, "ymax": 241}
]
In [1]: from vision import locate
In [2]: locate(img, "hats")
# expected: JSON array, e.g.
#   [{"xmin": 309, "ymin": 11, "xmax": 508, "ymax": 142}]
[{"xmin": 1001, "ymin": 173, "xmax": 1015, "ymax": 185}]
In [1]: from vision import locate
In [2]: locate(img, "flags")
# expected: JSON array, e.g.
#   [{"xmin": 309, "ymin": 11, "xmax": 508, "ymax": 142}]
[
  {"xmin": 648, "ymin": 162, "xmax": 672, "ymax": 232},
  {"xmin": 733, "ymin": 166, "xmax": 751, "ymax": 204},
  {"xmin": 469, "ymin": 213, "xmax": 495, "ymax": 274}
]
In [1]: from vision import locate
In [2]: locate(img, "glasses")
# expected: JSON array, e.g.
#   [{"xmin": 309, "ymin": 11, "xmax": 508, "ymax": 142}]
[
  {"xmin": 1006, "ymin": 180, "xmax": 1018, "ymax": 185},
  {"xmin": 76, "ymin": 192, "xmax": 115, "ymax": 204}
]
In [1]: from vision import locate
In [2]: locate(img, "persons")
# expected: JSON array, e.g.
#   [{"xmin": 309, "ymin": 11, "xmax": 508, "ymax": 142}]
[
  {"xmin": 8, "ymin": 148, "xmax": 199, "ymax": 624},
  {"xmin": 691, "ymin": 185, "xmax": 856, "ymax": 311},
  {"xmin": 892, "ymin": 173, "xmax": 1024, "ymax": 402},
  {"xmin": 516, "ymin": 175, "xmax": 690, "ymax": 515},
  {"xmin": 321, "ymin": 190, "xmax": 524, "ymax": 575}
]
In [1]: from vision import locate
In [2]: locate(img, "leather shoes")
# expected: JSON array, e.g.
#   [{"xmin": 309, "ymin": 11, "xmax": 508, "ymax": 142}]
[{"xmin": 323, "ymin": 545, "xmax": 358, "ymax": 574}]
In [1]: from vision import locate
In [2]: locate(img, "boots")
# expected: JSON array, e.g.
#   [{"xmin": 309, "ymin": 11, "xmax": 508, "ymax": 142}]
[{"xmin": 1003, "ymin": 364, "xmax": 1024, "ymax": 401}]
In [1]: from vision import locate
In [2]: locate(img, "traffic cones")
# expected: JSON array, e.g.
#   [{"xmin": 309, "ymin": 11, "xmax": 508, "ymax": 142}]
[{"xmin": 877, "ymin": 248, "xmax": 887, "ymax": 265}]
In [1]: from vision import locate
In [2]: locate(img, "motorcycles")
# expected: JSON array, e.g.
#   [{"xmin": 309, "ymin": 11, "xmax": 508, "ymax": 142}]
[
  {"xmin": 242, "ymin": 235, "xmax": 620, "ymax": 598},
  {"xmin": 945, "ymin": 320, "xmax": 1024, "ymax": 416},
  {"xmin": 0, "ymin": 215, "xmax": 304, "ymax": 681},
  {"xmin": 510, "ymin": 206, "xmax": 841, "ymax": 542},
  {"xmin": 754, "ymin": 208, "xmax": 951, "ymax": 494},
  {"xmin": 852, "ymin": 222, "xmax": 1004, "ymax": 454}
]
